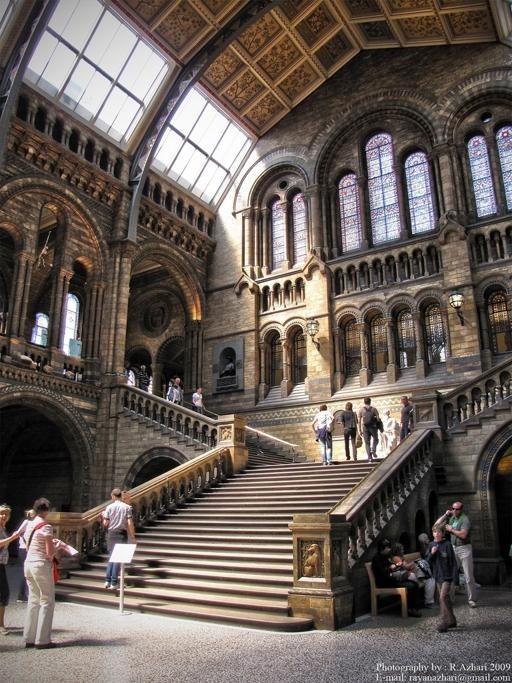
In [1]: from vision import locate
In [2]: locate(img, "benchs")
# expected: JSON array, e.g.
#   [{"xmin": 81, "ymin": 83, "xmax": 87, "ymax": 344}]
[{"xmin": 365, "ymin": 551, "xmax": 425, "ymax": 618}]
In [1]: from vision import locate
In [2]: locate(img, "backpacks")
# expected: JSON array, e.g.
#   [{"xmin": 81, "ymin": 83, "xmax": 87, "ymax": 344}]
[{"xmin": 362, "ymin": 407, "xmax": 374, "ymax": 426}]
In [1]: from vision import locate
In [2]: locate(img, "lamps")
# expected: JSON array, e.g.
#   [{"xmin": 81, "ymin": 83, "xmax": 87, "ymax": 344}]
[
  {"xmin": 305, "ymin": 317, "xmax": 320, "ymax": 351},
  {"xmin": 448, "ymin": 287, "xmax": 465, "ymax": 326}
]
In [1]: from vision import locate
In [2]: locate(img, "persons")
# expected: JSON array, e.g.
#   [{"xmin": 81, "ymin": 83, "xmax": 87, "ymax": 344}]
[
  {"xmin": 23, "ymin": 498, "xmax": 65, "ymax": 650},
  {"xmin": 400, "ymin": 394, "xmax": 414, "ymax": 438},
  {"xmin": 0, "ymin": 503, "xmax": 21, "ymax": 634},
  {"xmin": 102, "ymin": 488, "xmax": 136, "ymax": 587},
  {"xmin": 370, "ymin": 501, "xmax": 482, "ymax": 632},
  {"xmin": 311, "ymin": 404, "xmax": 336, "ymax": 464},
  {"xmin": 357, "ymin": 397, "xmax": 380, "ymax": 461},
  {"xmin": 191, "ymin": 386, "xmax": 206, "ymax": 414},
  {"xmin": 380, "ymin": 409, "xmax": 398, "ymax": 450},
  {"xmin": 124, "ymin": 361, "xmax": 183, "ymax": 407},
  {"xmin": 18, "ymin": 509, "xmax": 36, "ymax": 603},
  {"xmin": 338, "ymin": 400, "xmax": 359, "ymax": 460}
]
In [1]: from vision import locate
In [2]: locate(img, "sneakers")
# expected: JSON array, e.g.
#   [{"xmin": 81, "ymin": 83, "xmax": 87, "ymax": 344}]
[
  {"xmin": 104, "ymin": 581, "xmax": 120, "ymax": 590},
  {"xmin": 0, "ymin": 594, "xmax": 56, "ymax": 649},
  {"xmin": 323, "ymin": 448, "xmax": 378, "ymax": 465},
  {"xmin": 407, "ymin": 600, "xmax": 477, "ymax": 633}
]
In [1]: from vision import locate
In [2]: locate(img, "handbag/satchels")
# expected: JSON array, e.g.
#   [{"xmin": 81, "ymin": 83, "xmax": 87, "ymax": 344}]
[
  {"xmin": 52, "ymin": 561, "xmax": 59, "ymax": 584},
  {"xmin": 316, "ymin": 428, "xmax": 326, "ymax": 439},
  {"xmin": 391, "ymin": 568, "xmax": 408, "ymax": 581}
]
